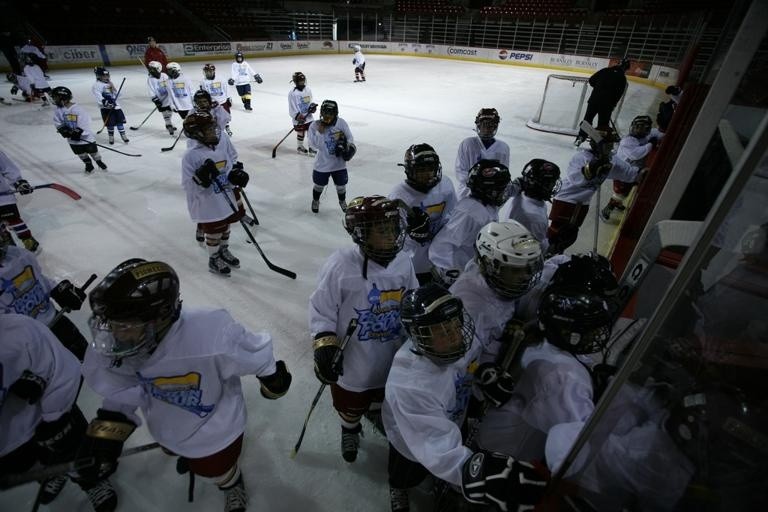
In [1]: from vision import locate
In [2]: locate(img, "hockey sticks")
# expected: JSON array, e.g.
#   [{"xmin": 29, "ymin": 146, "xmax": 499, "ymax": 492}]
[
  {"xmin": 294, "ymin": 318, "xmax": 358, "ymax": 452},
  {"xmin": 579, "ymin": 119, "xmax": 603, "ymax": 253},
  {"xmin": 97, "ymin": 77, "xmax": 126, "ymax": 133},
  {"xmin": 238, "ymin": 187, "xmax": 260, "ymax": 245},
  {"xmin": 129, "ymin": 106, "xmax": 158, "ymax": 130},
  {"xmin": 272, "ymin": 112, "xmax": 310, "ymax": 158},
  {"xmin": 214, "ymin": 176, "xmax": 296, "ymax": 280},
  {"xmin": 1, "ymin": 183, "xmax": 81, "ymax": 201},
  {"xmin": 77, "ymin": 136, "xmax": 142, "ymax": 157},
  {"xmin": 161, "ymin": 127, "xmax": 183, "ymax": 151}
]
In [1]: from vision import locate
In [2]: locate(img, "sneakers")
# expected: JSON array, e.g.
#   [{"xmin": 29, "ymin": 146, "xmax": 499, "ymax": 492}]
[
  {"xmin": 208, "ymin": 255, "xmax": 232, "ymax": 277},
  {"xmin": 22, "ymin": 238, "xmax": 40, "ymax": 254},
  {"xmin": 222, "ymin": 480, "xmax": 247, "ymax": 512},
  {"xmin": 84, "ymin": 162, "xmax": 94, "ymax": 173},
  {"xmin": 96, "ymin": 160, "xmax": 107, "ymax": 170},
  {"xmin": 311, "ymin": 199, "xmax": 321, "ymax": 215},
  {"xmin": 340, "ymin": 425, "xmax": 360, "ymax": 463},
  {"xmin": 85, "ymin": 479, "xmax": 118, "ymax": 512},
  {"xmin": 35, "ymin": 477, "xmax": 69, "ymax": 505},
  {"xmin": 354, "ymin": 79, "xmax": 359, "ymax": 82},
  {"xmin": 361, "ymin": 78, "xmax": 365, "ymax": 81},
  {"xmin": 220, "ymin": 246, "xmax": 239, "ymax": 266},
  {"xmin": 120, "ymin": 133, "xmax": 129, "ymax": 142},
  {"xmin": 109, "ymin": 135, "xmax": 114, "ymax": 144},
  {"xmin": 389, "ymin": 485, "xmax": 410, "ymax": 511},
  {"xmin": 296, "ymin": 147, "xmax": 308, "ymax": 155}
]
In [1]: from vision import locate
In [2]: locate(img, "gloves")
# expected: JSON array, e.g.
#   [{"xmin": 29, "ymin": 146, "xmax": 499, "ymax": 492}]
[
  {"xmin": 227, "ymin": 167, "xmax": 250, "ymax": 187},
  {"xmin": 253, "ymin": 73, "xmax": 263, "ymax": 84},
  {"xmin": 10, "ymin": 86, "xmax": 18, "ymax": 96},
  {"xmin": 462, "ymin": 448, "xmax": 550, "ymax": 510},
  {"xmin": 191, "ymin": 157, "xmax": 221, "ymax": 189},
  {"xmin": 48, "ymin": 278, "xmax": 88, "ymax": 311},
  {"xmin": 83, "ymin": 407, "xmax": 138, "ymax": 460},
  {"xmin": 312, "ymin": 330, "xmax": 344, "ymax": 386},
  {"xmin": 256, "ymin": 359, "xmax": 293, "ymax": 401},
  {"xmin": 309, "ymin": 104, "xmax": 316, "ymax": 113},
  {"xmin": 70, "ymin": 126, "xmax": 84, "ymax": 142},
  {"xmin": 13, "ymin": 178, "xmax": 34, "ymax": 196},
  {"xmin": 471, "ymin": 360, "xmax": 514, "ymax": 410}
]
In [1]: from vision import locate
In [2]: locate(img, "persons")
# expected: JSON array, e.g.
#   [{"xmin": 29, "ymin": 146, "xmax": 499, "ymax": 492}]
[
  {"xmin": 0, "ymin": 148, "xmax": 43, "ymax": 254},
  {"xmin": 352, "ymin": 45, "xmax": 368, "ymax": 82},
  {"xmin": 144, "ymin": 37, "xmax": 233, "ymax": 137},
  {"xmin": 76, "ymin": 257, "xmax": 293, "ymax": 511},
  {"xmin": 0, "ymin": 313, "xmax": 118, "ymax": 512},
  {"xmin": 308, "ymin": 100, "xmax": 678, "ymax": 511},
  {"xmin": 228, "ymin": 52, "xmax": 263, "ymax": 111},
  {"xmin": 288, "ymin": 72, "xmax": 318, "ymax": 157},
  {"xmin": 179, "ymin": 111, "xmax": 249, "ymax": 275},
  {"xmin": 556, "ymin": 222, "xmax": 768, "ymax": 511},
  {"xmin": 1, "ymin": 227, "xmax": 89, "ymax": 362},
  {"xmin": 574, "ymin": 58, "xmax": 632, "ymax": 151},
  {"xmin": 48, "ymin": 86, "xmax": 107, "ymax": 174},
  {"xmin": 92, "ymin": 65, "xmax": 130, "ymax": 145},
  {"xmin": 6, "ymin": 36, "xmax": 57, "ymax": 107}
]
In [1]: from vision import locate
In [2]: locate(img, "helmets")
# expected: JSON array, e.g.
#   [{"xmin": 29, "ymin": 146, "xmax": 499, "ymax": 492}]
[
  {"xmin": 628, "ymin": 114, "xmax": 653, "ymax": 139},
  {"xmin": 94, "ymin": 64, "xmax": 111, "ymax": 83},
  {"xmin": 148, "ymin": 53, "xmax": 243, "ymax": 134},
  {"xmin": 292, "ymin": 71, "xmax": 307, "ymax": 91},
  {"xmin": 51, "ymin": 86, "xmax": 73, "ymax": 108},
  {"xmin": 354, "ymin": 45, "xmax": 360, "ymax": 52},
  {"xmin": 319, "ymin": 99, "xmax": 339, "ymax": 127},
  {"xmin": 88, "ymin": 256, "xmax": 180, "ymax": 325}
]
